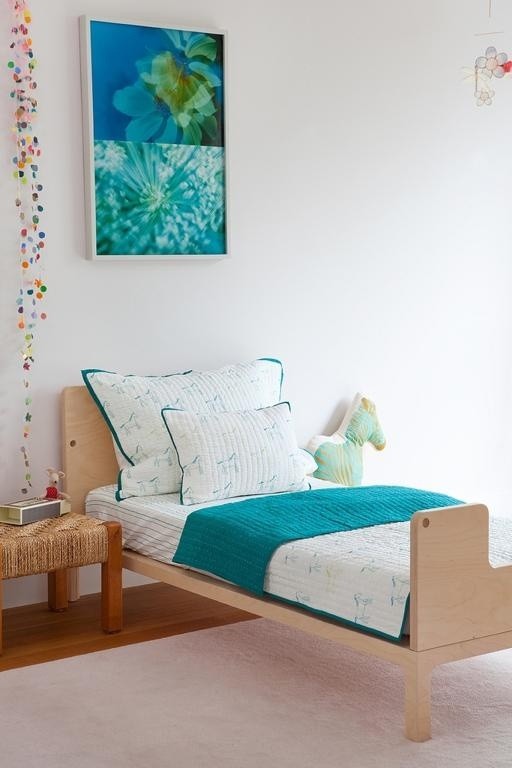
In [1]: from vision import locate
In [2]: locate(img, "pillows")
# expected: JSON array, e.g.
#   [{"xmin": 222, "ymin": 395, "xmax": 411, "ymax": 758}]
[{"xmin": 78, "ymin": 356, "xmax": 322, "ymax": 508}]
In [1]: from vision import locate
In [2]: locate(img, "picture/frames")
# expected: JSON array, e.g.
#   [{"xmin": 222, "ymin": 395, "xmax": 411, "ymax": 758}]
[{"xmin": 81, "ymin": 13, "xmax": 230, "ymax": 261}]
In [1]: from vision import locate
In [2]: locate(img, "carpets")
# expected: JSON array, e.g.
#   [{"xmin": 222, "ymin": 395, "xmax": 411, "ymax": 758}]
[{"xmin": 0, "ymin": 617, "xmax": 512, "ymax": 768}]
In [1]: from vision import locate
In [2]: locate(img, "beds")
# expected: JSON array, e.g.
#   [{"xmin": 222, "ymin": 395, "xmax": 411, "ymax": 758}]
[{"xmin": 57, "ymin": 384, "xmax": 509, "ymax": 741}]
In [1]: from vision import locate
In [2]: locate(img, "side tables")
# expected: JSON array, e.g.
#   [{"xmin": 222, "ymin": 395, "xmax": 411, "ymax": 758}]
[{"xmin": 0, "ymin": 512, "xmax": 123, "ymax": 637}]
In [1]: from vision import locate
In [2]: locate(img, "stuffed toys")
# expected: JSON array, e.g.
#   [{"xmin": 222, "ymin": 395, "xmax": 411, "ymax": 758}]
[{"xmin": 37, "ymin": 467, "xmax": 71, "ymax": 502}]
